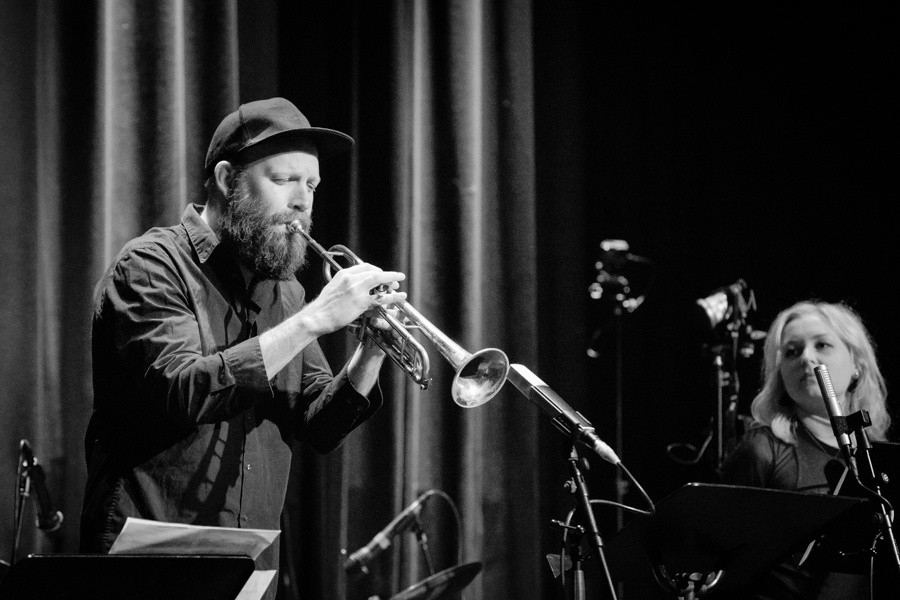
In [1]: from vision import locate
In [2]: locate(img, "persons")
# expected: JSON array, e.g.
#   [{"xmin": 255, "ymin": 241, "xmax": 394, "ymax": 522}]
[
  {"xmin": 720, "ymin": 301, "xmax": 892, "ymax": 495},
  {"xmin": 76, "ymin": 97, "xmax": 408, "ymax": 600}
]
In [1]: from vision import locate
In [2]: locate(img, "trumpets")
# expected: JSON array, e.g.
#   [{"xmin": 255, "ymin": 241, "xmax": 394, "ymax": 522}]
[{"xmin": 288, "ymin": 219, "xmax": 510, "ymax": 409}]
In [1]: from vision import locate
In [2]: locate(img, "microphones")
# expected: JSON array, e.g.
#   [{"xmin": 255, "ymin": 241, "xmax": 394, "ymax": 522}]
[
  {"xmin": 813, "ymin": 365, "xmax": 859, "ymax": 476},
  {"xmin": 342, "ymin": 490, "xmax": 433, "ymax": 580},
  {"xmin": 19, "ymin": 439, "xmax": 64, "ymax": 532},
  {"xmin": 507, "ymin": 363, "xmax": 621, "ymax": 465}
]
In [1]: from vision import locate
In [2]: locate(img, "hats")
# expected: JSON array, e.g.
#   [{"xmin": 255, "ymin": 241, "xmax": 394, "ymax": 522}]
[{"xmin": 204, "ymin": 97, "xmax": 356, "ymax": 181}]
